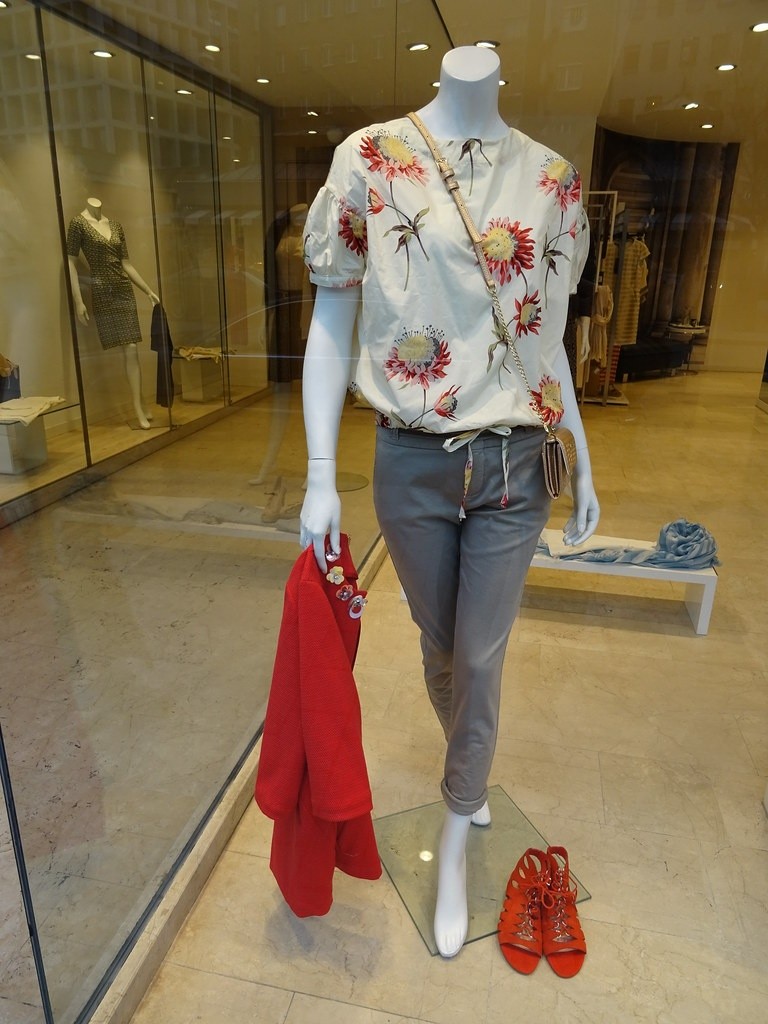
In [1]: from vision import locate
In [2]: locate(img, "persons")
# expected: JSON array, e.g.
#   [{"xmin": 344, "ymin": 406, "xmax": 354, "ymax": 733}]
[
  {"xmin": 66, "ymin": 197, "xmax": 162, "ymax": 428},
  {"xmin": 246, "ymin": 204, "xmax": 317, "ymax": 489},
  {"xmin": 301, "ymin": 48, "xmax": 598, "ymax": 953}
]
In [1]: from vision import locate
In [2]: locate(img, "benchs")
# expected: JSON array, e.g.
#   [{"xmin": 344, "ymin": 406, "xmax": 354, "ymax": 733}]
[{"xmin": 400, "ymin": 528, "xmax": 718, "ymax": 636}]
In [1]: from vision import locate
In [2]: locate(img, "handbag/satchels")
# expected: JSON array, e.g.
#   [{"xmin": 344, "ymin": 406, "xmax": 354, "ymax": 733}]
[{"xmin": 541, "ymin": 427, "xmax": 578, "ymax": 501}]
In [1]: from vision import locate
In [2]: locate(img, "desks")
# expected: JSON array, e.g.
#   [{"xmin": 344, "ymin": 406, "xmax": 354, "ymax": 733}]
[
  {"xmin": 172, "ymin": 350, "xmax": 237, "ymax": 401},
  {"xmin": 0, "ymin": 402, "xmax": 80, "ymax": 476}
]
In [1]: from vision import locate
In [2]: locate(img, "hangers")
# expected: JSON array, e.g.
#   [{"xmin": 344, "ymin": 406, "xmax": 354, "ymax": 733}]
[{"xmin": 626, "ymin": 232, "xmax": 648, "ymax": 244}]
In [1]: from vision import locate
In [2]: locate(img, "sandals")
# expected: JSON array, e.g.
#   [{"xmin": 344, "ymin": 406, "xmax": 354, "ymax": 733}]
[
  {"xmin": 496, "ymin": 847, "xmax": 555, "ymax": 976},
  {"xmin": 541, "ymin": 845, "xmax": 586, "ymax": 979}
]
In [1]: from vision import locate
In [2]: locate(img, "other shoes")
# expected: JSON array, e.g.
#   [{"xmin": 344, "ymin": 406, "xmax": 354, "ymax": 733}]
[{"xmin": 602, "ymin": 382, "xmax": 622, "ymax": 398}]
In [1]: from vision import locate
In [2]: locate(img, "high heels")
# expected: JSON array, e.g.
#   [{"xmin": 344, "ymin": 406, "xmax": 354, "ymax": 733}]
[
  {"xmin": 279, "ymin": 500, "xmax": 303, "ymax": 520},
  {"xmin": 260, "ymin": 476, "xmax": 288, "ymax": 524}
]
